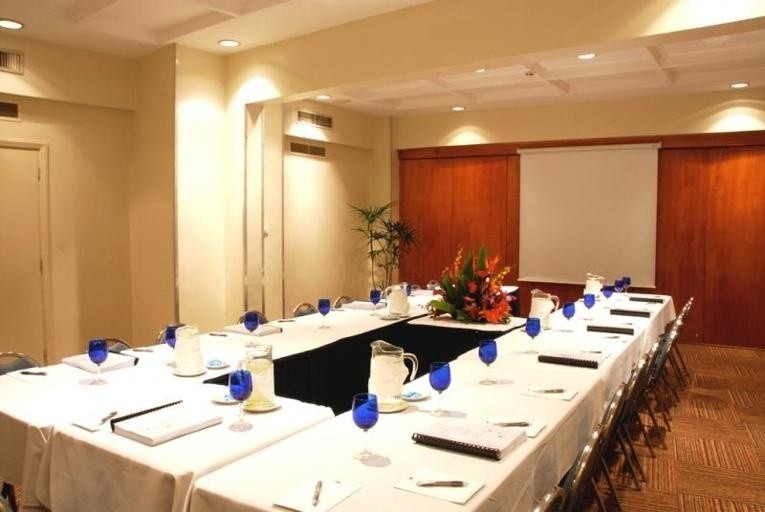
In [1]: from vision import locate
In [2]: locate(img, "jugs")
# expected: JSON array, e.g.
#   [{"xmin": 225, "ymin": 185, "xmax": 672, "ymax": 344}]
[
  {"xmin": 527, "ymin": 286, "xmax": 559, "ymax": 332},
  {"xmin": 381, "ymin": 281, "xmax": 410, "ymax": 321},
  {"xmin": 369, "ymin": 337, "xmax": 418, "ymax": 414},
  {"xmin": 243, "ymin": 342, "xmax": 284, "ymax": 414},
  {"xmin": 173, "ymin": 321, "xmax": 208, "ymax": 376}
]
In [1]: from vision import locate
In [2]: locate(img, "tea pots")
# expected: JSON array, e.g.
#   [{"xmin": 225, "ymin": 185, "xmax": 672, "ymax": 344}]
[{"xmin": 583, "ymin": 272, "xmax": 605, "ymax": 298}]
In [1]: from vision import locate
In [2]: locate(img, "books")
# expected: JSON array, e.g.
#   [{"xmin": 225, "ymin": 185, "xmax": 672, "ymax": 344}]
[
  {"xmin": 610, "ymin": 305, "xmax": 650, "ymax": 318},
  {"xmin": 110, "ymin": 400, "xmax": 223, "ymax": 446},
  {"xmin": 342, "ymin": 299, "xmax": 387, "ymax": 310},
  {"xmin": 630, "ymin": 295, "xmax": 664, "ymax": 304},
  {"xmin": 537, "ymin": 348, "xmax": 604, "ymax": 369},
  {"xmin": 62, "ymin": 350, "xmax": 139, "ymax": 373},
  {"xmin": 587, "ymin": 321, "xmax": 634, "ymax": 335},
  {"xmin": 223, "ymin": 323, "xmax": 283, "ymax": 336},
  {"xmin": 411, "ymin": 413, "xmax": 527, "ymax": 460}
]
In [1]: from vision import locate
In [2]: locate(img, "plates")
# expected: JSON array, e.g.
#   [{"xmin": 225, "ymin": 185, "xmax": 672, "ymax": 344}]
[
  {"xmin": 205, "ymin": 357, "xmax": 231, "ymax": 369},
  {"xmin": 401, "ymin": 387, "xmax": 430, "ymax": 402}
]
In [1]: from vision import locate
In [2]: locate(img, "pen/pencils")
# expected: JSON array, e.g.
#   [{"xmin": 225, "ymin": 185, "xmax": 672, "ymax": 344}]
[
  {"xmin": 493, "ymin": 422, "xmax": 530, "ymax": 426},
  {"xmin": 312, "ymin": 480, "xmax": 322, "ymax": 506},
  {"xmin": 331, "ymin": 309, "xmax": 344, "ymax": 311},
  {"xmin": 210, "ymin": 333, "xmax": 228, "ymax": 336},
  {"xmin": 103, "ymin": 411, "xmax": 118, "ymax": 422},
  {"xmin": 278, "ymin": 320, "xmax": 295, "ymax": 322},
  {"xmin": 622, "ymin": 322, "xmax": 632, "ymax": 325},
  {"xmin": 532, "ymin": 389, "xmax": 564, "ymax": 393},
  {"xmin": 418, "ymin": 480, "xmax": 464, "ymax": 486},
  {"xmin": 22, "ymin": 372, "xmax": 47, "ymax": 375},
  {"xmin": 133, "ymin": 348, "xmax": 154, "ymax": 352},
  {"xmin": 608, "ymin": 336, "xmax": 619, "ymax": 338},
  {"xmin": 589, "ymin": 350, "xmax": 602, "ymax": 353}
]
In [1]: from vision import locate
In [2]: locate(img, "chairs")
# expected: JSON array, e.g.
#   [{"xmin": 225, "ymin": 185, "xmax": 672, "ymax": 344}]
[{"xmin": 0, "ymin": 278, "xmax": 695, "ymax": 511}]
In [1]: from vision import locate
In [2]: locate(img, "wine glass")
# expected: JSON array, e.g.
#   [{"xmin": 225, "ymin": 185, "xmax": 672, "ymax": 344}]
[
  {"xmin": 84, "ymin": 339, "xmax": 110, "ymax": 385},
  {"xmin": 584, "ymin": 294, "xmax": 596, "ymax": 323},
  {"xmin": 242, "ymin": 312, "xmax": 260, "ymax": 347},
  {"xmin": 226, "ymin": 368, "xmax": 255, "ymax": 431},
  {"xmin": 369, "ymin": 289, "xmax": 381, "ymax": 316},
  {"xmin": 430, "ymin": 359, "xmax": 451, "ymax": 418},
  {"xmin": 349, "ymin": 393, "xmax": 378, "ymax": 434},
  {"xmin": 613, "ymin": 275, "xmax": 631, "ymax": 300},
  {"xmin": 560, "ymin": 302, "xmax": 577, "ymax": 332},
  {"xmin": 525, "ymin": 317, "xmax": 541, "ymax": 355},
  {"xmin": 601, "ymin": 279, "xmax": 613, "ymax": 310},
  {"xmin": 165, "ymin": 325, "xmax": 177, "ymax": 351},
  {"xmin": 318, "ymin": 298, "xmax": 332, "ymax": 331},
  {"xmin": 476, "ymin": 339, "xmax": 498, "ymax": 385}
]
